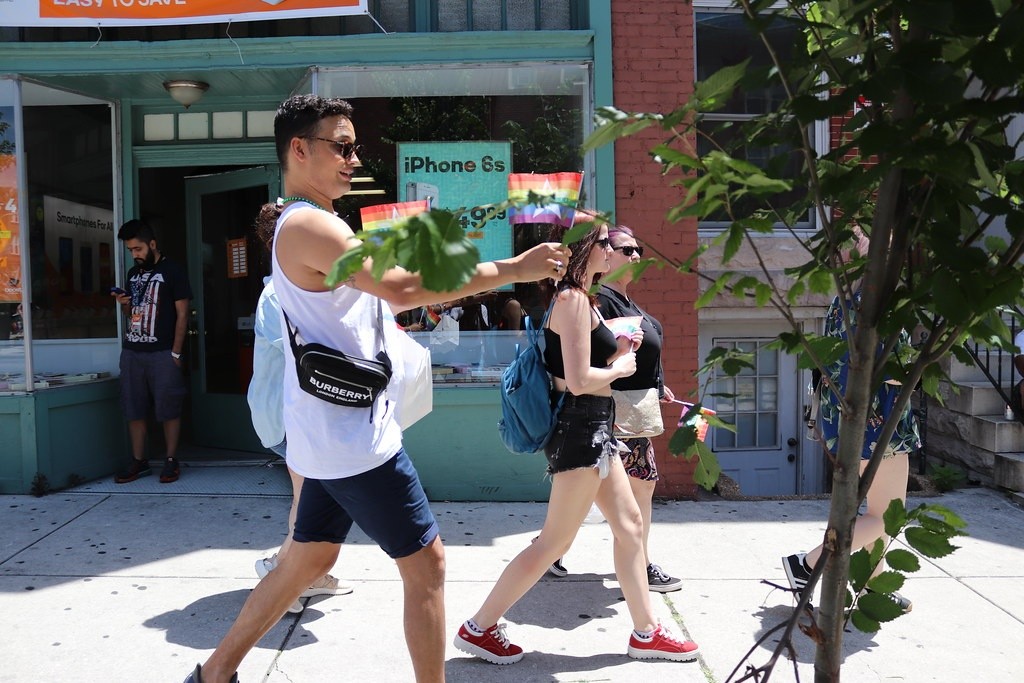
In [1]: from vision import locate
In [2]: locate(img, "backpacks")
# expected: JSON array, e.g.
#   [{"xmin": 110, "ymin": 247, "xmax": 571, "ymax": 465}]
[{"xmin": 497, "ymin": 286, "xmax": 592, "ymax": 456}]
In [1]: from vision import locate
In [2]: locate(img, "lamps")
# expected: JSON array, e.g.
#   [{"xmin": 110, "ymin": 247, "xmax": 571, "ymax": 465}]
[{"xmin": 163, "ymin": 81, "xmax": 209, "ymax": 109}]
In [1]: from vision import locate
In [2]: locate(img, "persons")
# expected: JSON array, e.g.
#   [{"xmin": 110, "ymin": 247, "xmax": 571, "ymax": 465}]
[
  {"xmin": 779, "ymin": 224, "xmax": 923, "ymax": 615},
  {"xmin": 181, "ymin": 94, "xmax": 574, "ymax": 683},
  {"xmin": 531, "ymin": 225, "xmax": 684, "ymax": 590},
  {"xmin": 111, "ymin": 219, "xmax": 188, "ymax": 484},
  {"xmin": 451, "ymin": 209, "xmax": 699, "ymax": 663}
]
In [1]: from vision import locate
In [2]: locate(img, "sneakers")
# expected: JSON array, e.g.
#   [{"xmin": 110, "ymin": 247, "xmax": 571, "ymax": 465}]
[
  {"xmin": 255, "ymin": 553, "xmax": 304, "ymax": 613},
  {"xmin": 181, "ymin": 663, "xmax": 240, "ymax": 683},
  {"xmin": 453, "ymin": 619, "xmax": 524, "ymax": 665},
  {"xmin": 855, "ymin": 586, "xmax": 913, "ymax": 615},
  {"xmin": 531, "ymin": 535, "xmax": 567, "ymax": 577},
  {"xmin": 300, "ymin": 573, "xmax": 354, "ymax": 598},
  {"xmin": 628, "ymin": 622, "xmax": 700, "ymax": 661},
  {"xmin": 782, "ymin": 553, "xmax": 817, "ymax": 616},
  {"xmin": 646, "ymin": 563, "xmax": 682, "ymax": 592}
]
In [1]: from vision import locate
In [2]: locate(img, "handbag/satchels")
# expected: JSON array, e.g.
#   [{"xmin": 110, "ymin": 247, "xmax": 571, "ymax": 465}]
[{"xmin": 295, "ymin": 343, "xmax": 393, "ymax": 425}]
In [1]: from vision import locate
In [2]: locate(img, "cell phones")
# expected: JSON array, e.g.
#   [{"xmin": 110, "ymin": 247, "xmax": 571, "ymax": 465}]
[{"xmin": 111, "ymin": 287, "xmax": 130, "ymax": 298}]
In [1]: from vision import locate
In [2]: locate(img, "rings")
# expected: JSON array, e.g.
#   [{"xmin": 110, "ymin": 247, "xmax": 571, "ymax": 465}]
[{"xmin": 556, "ymin": 260, "xmax": 564, "ymax": 271}]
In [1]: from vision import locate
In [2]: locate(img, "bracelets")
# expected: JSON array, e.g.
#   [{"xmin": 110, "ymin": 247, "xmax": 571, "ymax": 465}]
[{"xmin": 170, "ymin": 352, "xmax": 180, "ymax": 358}]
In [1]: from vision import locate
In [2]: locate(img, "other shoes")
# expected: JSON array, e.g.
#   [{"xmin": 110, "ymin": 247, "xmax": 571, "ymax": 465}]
[
  {"xmin": 159, "ymin": 456, "xmax": 180, "ymax": 483},
  {"xmin": 114, "ymin": 457, "xmax": 152, "ymax": 483}
]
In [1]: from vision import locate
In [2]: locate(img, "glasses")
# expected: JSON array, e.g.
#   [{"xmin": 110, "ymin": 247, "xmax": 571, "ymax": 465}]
[
  {"xmin": 595, "ymin": 236, "xmax": 610, "ymax": 247},
  {"xmin": 303, "ymin": 136, "xmax": 365, "ymax": 159},
  {"xmin": 614, "ymin": 246, "xmax": 644, "ymax": 257}
]
